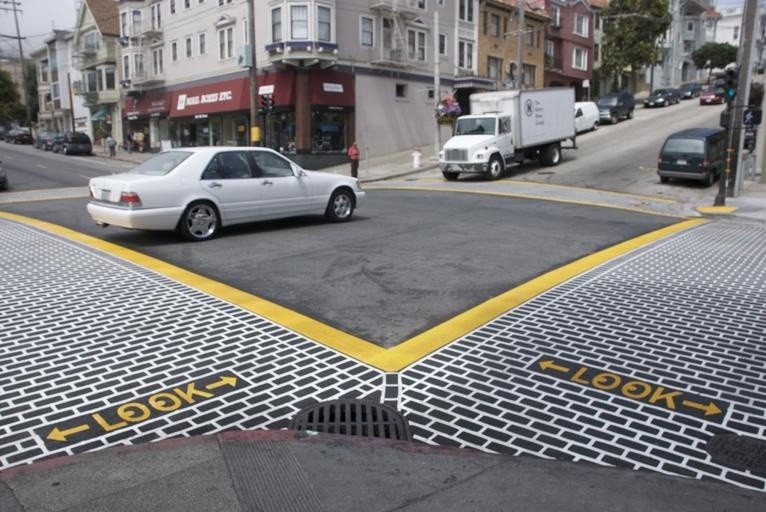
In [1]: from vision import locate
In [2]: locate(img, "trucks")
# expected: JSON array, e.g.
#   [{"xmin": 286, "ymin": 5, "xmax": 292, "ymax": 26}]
[{"xmin": 439, "ymin": 85, "xmax": 575, "ymax": 180}]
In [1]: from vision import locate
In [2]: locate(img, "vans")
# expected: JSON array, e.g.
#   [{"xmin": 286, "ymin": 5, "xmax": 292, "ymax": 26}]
[{"xmin": 657, "ymin": 127, "xmax": 726, "ymax": 186}]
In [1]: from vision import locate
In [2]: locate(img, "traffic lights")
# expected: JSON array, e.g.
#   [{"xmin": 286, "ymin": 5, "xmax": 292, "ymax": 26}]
[
  {"xmin": 259, "ymin": 95, "xmax": 268, "ymax": 114},
  {"xmin": 269, "ymin": 95, "xmax": 275, "ymax": 111}
]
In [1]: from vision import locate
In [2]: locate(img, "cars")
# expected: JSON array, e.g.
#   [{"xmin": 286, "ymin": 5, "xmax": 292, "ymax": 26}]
[
  {"xmin": 575, "ymin": 92, "xmax": 635, "ymax": 133},
  {"xmin": 0, "ymin": 160, "xmax": 8, "ymax": 191},
  {"xmin": 86, "ymin": 145, "xmax": 365, "ymax": 242},
  {"xmin": 35, "ymin": 131, "xmax": 91, "ymax": 154},
  {"xmin": 0, "ymin": 128, "xmax": 33, "ymax": 144},
  {"xmin": 644, "ymin": 83, "xmax": 725, "ymax": 107}
]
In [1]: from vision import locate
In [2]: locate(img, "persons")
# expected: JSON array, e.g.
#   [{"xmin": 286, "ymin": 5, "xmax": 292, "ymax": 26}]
[
  {"xmin": 135, "ymin": 129, "xmax": 144, "ymax": 152},
  {"xmin": 126, "ymin": 130, "xmax": 135, "ymax": 154},
  {"xmin": 348, "ymin": 143, "xmax": 360, "ymax": 178},
  {"xmin": 107, "ymin": 135, "xmax": 116, "ymax": 156}
]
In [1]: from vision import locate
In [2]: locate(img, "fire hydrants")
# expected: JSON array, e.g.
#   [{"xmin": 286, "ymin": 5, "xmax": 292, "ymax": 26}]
[{"xmin": 412, "ymin": 146, "xmax": 422, "ymax": 169}]
